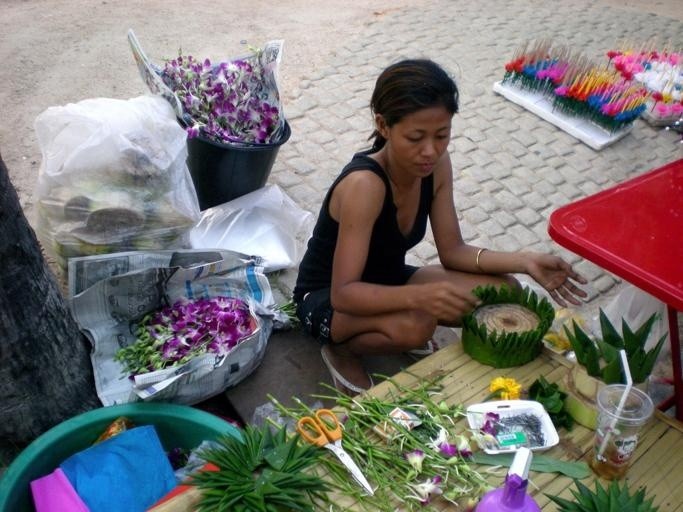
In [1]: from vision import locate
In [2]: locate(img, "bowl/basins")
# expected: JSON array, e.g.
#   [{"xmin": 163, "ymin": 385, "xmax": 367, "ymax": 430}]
[{"xmin": 1, "ymin": 401, "xmax": 251, "ymax": 511}]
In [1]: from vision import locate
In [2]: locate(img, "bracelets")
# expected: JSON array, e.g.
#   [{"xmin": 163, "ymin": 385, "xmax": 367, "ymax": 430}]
[{"xmin": 475, "ymin": 248, "xmax": 488, "ymax": 273}]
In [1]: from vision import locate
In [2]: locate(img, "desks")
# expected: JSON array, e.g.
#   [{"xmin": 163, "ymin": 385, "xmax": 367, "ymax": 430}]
[{"xmin": 549, "ymin": 160, "xmax": 681, "ymax": 420}]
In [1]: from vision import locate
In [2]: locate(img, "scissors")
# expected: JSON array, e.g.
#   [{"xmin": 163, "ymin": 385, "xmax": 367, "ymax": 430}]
[{"xmin": 298, "ymin": 409, "xmax": 374, "ymax": 495}]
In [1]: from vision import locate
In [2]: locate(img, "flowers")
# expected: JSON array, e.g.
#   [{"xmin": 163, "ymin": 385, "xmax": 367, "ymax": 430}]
[
  {"xmin": 105, "ymin": 290, "xmax": 298, "ymax": 376},
  {"xmin": 123, "ymin": 28, "xmax": 285, "ymax": 146}
]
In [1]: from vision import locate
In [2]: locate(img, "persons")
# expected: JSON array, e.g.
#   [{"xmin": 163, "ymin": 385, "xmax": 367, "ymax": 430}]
[{"xmin": 293, "ymin": 56, "xmax": 589, "ymax": 397}]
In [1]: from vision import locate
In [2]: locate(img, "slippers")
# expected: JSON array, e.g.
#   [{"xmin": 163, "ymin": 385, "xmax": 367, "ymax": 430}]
[
  {"xmin": 407, "ymin": 340, "xmax": 435, "ymax": 362},
  {"xmin": 320, "ymin": 347, "xmax": 374, "ymax": 397}
]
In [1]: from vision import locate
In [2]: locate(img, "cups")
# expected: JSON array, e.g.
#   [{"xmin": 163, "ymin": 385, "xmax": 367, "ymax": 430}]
[{"xmin": 589, "ymin": 383, "xmax": 655, "ymax": 481}]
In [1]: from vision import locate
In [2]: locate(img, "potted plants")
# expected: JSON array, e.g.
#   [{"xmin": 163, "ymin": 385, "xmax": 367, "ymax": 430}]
[{"xmin": 562, "ymin": 307, "xmax": 670, "ymax": 403}]
